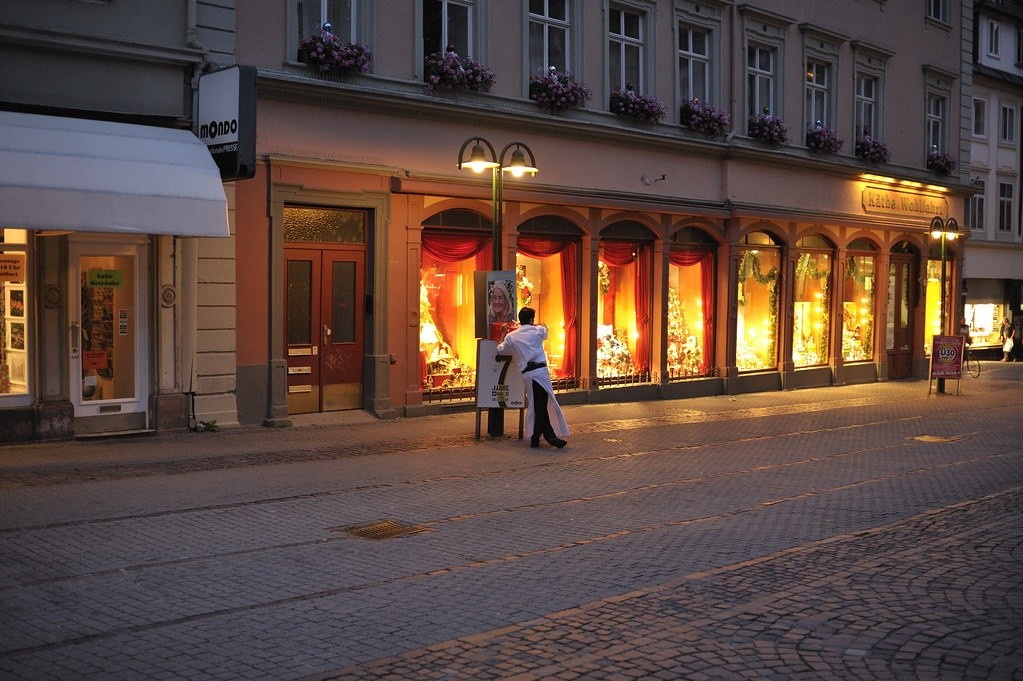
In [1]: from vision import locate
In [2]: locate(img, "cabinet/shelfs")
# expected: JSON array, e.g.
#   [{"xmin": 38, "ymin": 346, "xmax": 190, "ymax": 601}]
[{"xmin": 87, "ymin": 277, "xmax": 127, "ymax": 399}]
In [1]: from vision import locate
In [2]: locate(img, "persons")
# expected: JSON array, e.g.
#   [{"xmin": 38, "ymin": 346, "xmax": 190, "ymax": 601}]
[
  {"xmin": 489, "ymin": 282, "xmax": 513, "ymax": 331},
  {"xmin": 997, "ymin": 318, "xmax": 1017, "ymax": 361},
  {"xmin": 960, "ymin": 318, "xmax": 969, "ymax": 370},
  {"xmin": 498, "ymin": 307, "xmax": 570, "ymax": 448}
]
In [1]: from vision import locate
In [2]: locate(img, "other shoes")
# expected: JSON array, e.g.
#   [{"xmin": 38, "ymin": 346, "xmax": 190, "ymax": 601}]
[
  {"xmin": 549, "ymin": 438, "xmax": 567, "ymax": 448},
  {"xmin": 531, "ymin": 442, "xmax": 540, "ymax": 447}
]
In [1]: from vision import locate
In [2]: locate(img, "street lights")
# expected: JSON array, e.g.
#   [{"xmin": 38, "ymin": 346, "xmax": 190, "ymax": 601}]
[
  {"xmin": 923, "ymin": 216, "xmax": 965, "ymax": 395},
  {"xmin": 454, "ymin": 135, "xmax": 540, "ymax": 437}
]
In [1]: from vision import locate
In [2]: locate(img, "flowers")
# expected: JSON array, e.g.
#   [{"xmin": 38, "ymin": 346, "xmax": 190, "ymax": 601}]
[
  {"xmin": 678, "ymin": 101, "xmax": 732, "ymax": 142},
  {"xmin": 928, "ymin": 150, "xmax": 959, "ymax": 177},
  {"xmin": 806, "ymin": 126, "xmax": 847, "ymax": 154},
  {"xmin": 855, "ymin": 135, "xmax": 893, "ymax": 163},
  {"xmin": 297, "ymin": 28, "xmax": 373, "ymax": 76},
  {"xmin": 610, "ymin": 89, "xmax": 668, "ymax": 122},
  {"xmin": 424, "ymin": 51, "xmax": 498, "ymax": 92},
  {"xmin": 745, "ymin": 113, "xmax": 793, "ymax": 150},
  {"xmin": 526, "ymin": 70, "xmax": 594, "ymax": 107}
]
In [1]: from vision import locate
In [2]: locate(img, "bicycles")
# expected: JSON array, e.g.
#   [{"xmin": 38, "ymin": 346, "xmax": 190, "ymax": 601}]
[{"xmin": 961, "ymin": 340, "xmax": 981, "ymax": 379}]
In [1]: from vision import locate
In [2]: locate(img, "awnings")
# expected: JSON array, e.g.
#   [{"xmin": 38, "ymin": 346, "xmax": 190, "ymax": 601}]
[{"xmin": 0, "ymin": 110, "xmax": 230, "ymax": 238}]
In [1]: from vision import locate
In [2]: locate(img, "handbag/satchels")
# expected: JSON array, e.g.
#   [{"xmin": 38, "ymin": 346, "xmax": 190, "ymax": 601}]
[{"xmin": 1002, "ymin": 338, "xmax": 1014, "ymax": 352}]
